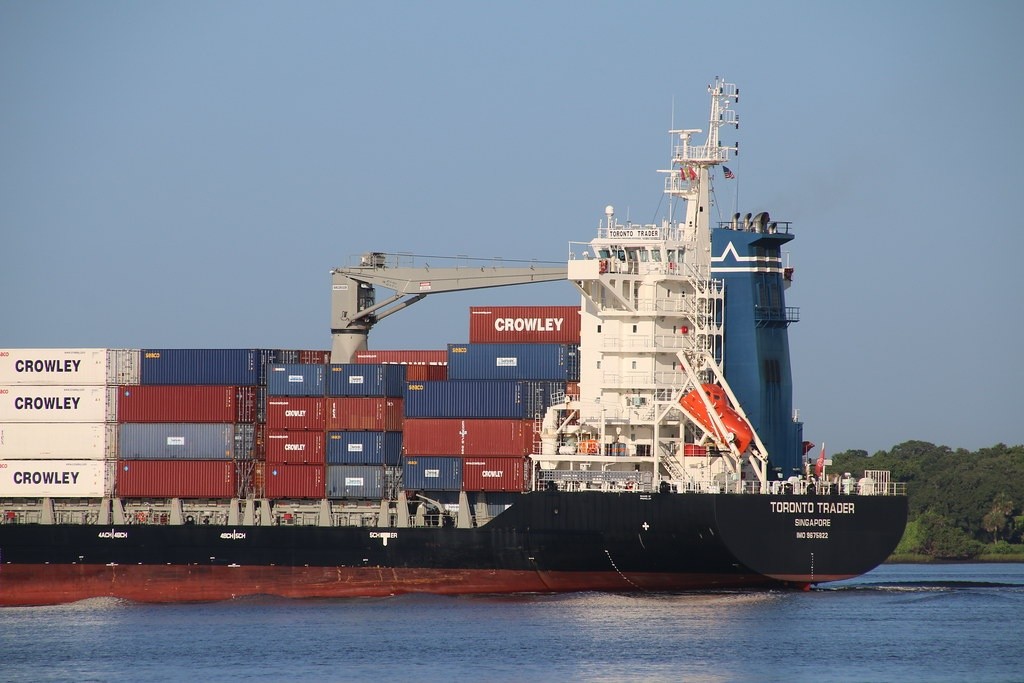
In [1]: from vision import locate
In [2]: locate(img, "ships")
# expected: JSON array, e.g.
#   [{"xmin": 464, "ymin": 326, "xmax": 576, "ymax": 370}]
[{"xmin": 0, "ymin": 74, "xmax": 909, "ymax": 609}]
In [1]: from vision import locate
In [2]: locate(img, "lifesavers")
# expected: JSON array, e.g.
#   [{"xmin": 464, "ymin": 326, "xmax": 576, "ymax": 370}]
[
  {"xmin": 136, "ymin": 512, "xmax": 146, "ymax": 521},
  {"xmin": 589, "ymin": 438, "xmax": 597, "ymax": 454}
]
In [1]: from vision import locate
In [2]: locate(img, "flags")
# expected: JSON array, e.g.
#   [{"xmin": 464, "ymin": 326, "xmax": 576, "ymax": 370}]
[
  {"xmin": 723, "ymin": 165, "xmax": 735, "ymax": 179},
  {"xmin": 814, "ymin": 442, "xmax": 825, "ymax": 477},
  {"xmin": 679, "ymin": 165, "xmax": 698, "ymax": 180}
]
